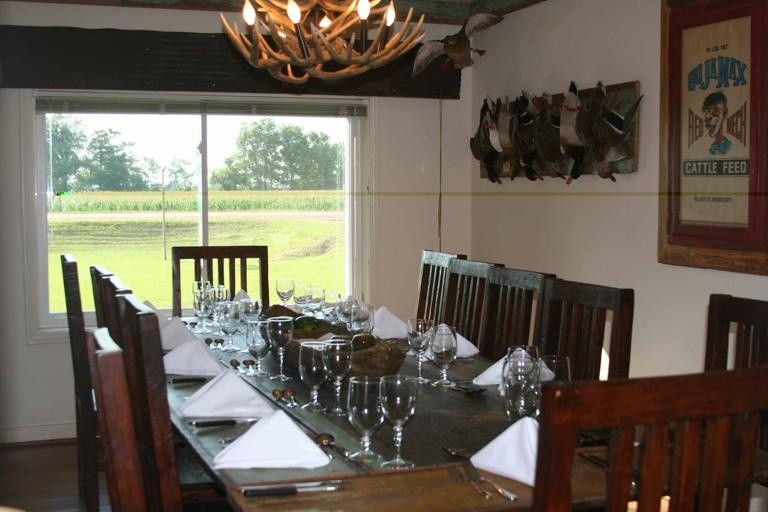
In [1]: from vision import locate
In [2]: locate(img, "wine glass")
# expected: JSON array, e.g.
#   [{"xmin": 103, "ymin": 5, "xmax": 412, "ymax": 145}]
[
  {"xmin": 275, "ymin": 278, "xmax": 375, "ymax": 337},
  {"xmin": 321, "ymin": 337, "xmax": 354, "ymax": 416},
  {"xmin": 246, "ymin": 319, "xmax": 272, "ymax": 377},
  {"xmin": 265, "ymin": 317, "xmax": 296, "ymax": 383},
  {"xmin": 377, "ymin": 375, "xmax": 418, "ymax": 470},
  {"xmin": 406, "ymin": 318, "xmax": 436, "ymax": 385},
  {"xmin": 427, "ymin": 324, "xmax": 459, "ymax": 389},
  {"xmin": 343, "ymin": 376, "xmax": 386, "ymax": 464},
  {"xmin": 295, "ymin": 341, "xmax": 332, "ymax": 413},
  {"xmin": 189, "ymin": 280, "xmax": 265, "ymax": 354}
]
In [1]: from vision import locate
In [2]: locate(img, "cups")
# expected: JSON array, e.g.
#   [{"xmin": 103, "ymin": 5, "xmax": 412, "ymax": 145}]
[{"xmin": 500, "ymin": 347, "xmax": 573, "ymax": 423}]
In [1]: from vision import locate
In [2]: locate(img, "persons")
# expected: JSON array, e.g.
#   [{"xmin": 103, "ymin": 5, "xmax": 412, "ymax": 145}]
[{"xmin": 698, "ymin": 92, "xmax": 732, "ymax": 156}]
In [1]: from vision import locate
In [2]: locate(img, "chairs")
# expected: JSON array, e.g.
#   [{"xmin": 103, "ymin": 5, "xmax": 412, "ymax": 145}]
[
  {"xmin": 171, "ymin": 246, "xmax": 270, "ymax": 317},
  {"xmin": 442, "ymin": 258, "xmax": 505, "ymax": 357},
  {"xmin": 85, "ymin": 328, "xmax": 149, "ymax": 512},
  {"xmin": 115, "ymin": 293, "xmax": 226, "ymax": 510},
  {"xmin": 533, "ymin": 365, "xmax": 766, "ymax": 511},
  {"xmin": 102, "ymin": 277, "xmax": 133, "ymax": 343},
  {"xmin": 477, "ymin": 266, "xmax": 556, "ymax": 362},
  {"xmin": 416, "ymin": 249, "xmax": 467, "ymax": 335},
  {"xmin": 89, "ymin": 266, "xmax": 113, "ymax": 329},
  {"xmin": 589, "ymin": 293, "xmax": 768, "ymax": 512},
  {"xmin": 539, "ymin": 277, "xmax": 635, "ymax": 382},
  {"xmin": 59, "ymin": 254, "xmax": 105, "ymax": 512}
]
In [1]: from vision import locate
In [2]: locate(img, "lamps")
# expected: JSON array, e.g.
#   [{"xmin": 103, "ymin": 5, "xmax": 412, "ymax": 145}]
[{"xmin": 219, "ymin": 0, "xmax": 427, "ymax": 83}]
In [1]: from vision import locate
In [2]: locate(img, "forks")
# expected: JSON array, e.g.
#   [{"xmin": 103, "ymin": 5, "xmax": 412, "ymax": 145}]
[{"xmin": 440, "ymin": 444, "xmax": 519, "ymax": 502}]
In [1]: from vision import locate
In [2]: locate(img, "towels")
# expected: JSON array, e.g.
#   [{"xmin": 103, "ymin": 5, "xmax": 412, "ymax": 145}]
[
  {"xmin": 407, "ymin": 322, "xmax": 480, "ymax": 361},
  {"xmin": 213, "ymin": 407, "xmax": 331, "ymax": 474},
  {"xmin": 371, "ymin": 305, "xmax": 414, "ymax": 340},
  {"xmin": 471, "ymin": 415, "xmax": 539, "ymax": 487},
  {"xmin": 145, "ymin": 299, "xmax": 168, "ymax": 327},
  {"xmin": 177, "ymin": 367, "xmax": 276, "ymax": 421},
  {"xmin": 472, "ymin": 352, "xmax": 557, "ymax": 385},
  {"xmin": 161, "ymin": 317, "xmax": 199, "ymax": 351},
  {"xmin": 162, "ymin": 339, "xmax": 225, "ymax": 379}
]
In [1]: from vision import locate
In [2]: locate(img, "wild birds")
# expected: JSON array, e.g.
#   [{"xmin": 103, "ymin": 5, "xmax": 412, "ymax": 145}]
[
  {"xmin": 469, "ymin": 80, "xmax": 645, "ymax": 185},
  {"xmin": 411, "ymin": 13, "xmax": 504, "ymax": 80}
]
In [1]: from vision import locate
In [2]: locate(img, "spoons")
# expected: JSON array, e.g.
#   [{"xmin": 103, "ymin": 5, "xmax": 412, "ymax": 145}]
[
  {"xmin": 271, "ymin": 386, "xmax": 302, "ymax": 409},
  {"xmin": 315, "ymin": 432, "xmax": 354, "ymax": 458},
  {"xmin": 230, "ymin": 358, "xmax": 256, "ymax": 375}
]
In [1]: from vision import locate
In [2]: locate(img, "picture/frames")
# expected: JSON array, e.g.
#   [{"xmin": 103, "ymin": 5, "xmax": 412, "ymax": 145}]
[{"xmin": 657, "ymin": 0, "xmax": 768, "ymax": 275}]
